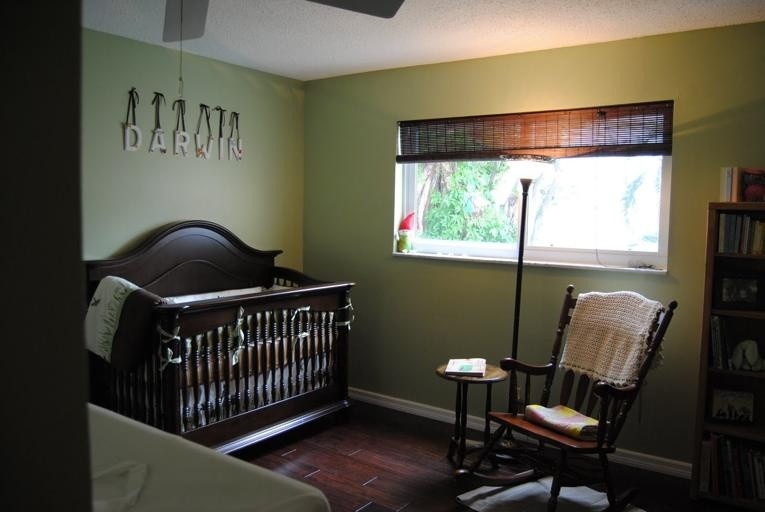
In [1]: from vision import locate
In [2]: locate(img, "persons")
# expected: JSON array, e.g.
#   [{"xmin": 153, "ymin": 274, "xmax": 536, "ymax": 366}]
[{"xmin": 395, "ymin": 212, "xmax": 416, "ymax": 255}]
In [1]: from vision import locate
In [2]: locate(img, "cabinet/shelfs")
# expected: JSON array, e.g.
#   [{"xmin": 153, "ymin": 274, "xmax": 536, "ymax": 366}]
[{"xmin": 687, "ymin": 198, "xmax": 764, "ymax": 511}]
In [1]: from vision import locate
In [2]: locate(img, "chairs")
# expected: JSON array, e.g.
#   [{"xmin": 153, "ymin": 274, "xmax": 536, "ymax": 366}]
[{"xmin": 462, "ymin": 282, "xmax": 678, "ymax": 510}]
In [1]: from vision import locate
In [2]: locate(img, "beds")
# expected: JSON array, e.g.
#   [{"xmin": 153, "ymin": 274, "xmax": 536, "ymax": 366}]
[
  {"xmin": 82, "ymin": 219, "xmax": 356, "ymax": 456},
  {"xmin": 88, "ymin": 403, "xmax": 330, "ymax": 510}
]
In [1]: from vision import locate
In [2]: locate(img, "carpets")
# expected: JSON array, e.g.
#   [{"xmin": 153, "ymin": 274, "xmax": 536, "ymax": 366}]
[{"xmin": 455, "ymin": 474, "xmax": 613, "ymax": 509}]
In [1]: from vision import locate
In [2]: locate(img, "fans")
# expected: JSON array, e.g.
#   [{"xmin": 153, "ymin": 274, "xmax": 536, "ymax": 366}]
[{"xmin": 162, "ymin": 1, "xmax": 405, "ymax": 97}]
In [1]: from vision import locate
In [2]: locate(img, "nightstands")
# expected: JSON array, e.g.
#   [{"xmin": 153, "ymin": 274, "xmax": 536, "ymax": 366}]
[{"xmin": 435, "ymin": 360, "xmax": 509, "ymax": 473}]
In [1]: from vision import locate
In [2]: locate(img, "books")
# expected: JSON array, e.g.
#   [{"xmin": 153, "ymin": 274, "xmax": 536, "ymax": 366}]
[
  {"xmin": 445, "ymin": 356, "xmax": 487, "ymax": 378},
  {"xmin": 695, "ymin": 165, "xmax": 764, "ymax": 505}
]
